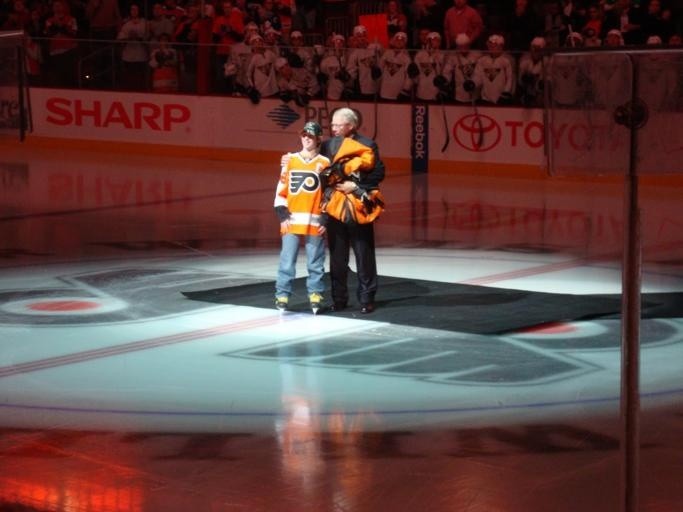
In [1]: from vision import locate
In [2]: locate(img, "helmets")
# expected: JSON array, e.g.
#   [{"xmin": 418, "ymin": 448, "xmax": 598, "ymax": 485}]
[
  {"xmin": 488, "ymin": 33, "xmax": 505, "ymax": 46},
  {"xmin": 426, "ymin": 32, "xmax": 440, "ymax": 53},
  {"xmin": 647, "ymin": 36, "xmax": 661, "ymax": 45},
  {"xmin": 567, "ymin": 32, "xmax": 583, "ymax": 43},
  {"xmin": 531, "ymin": 36, "xmax": 546, "ymax": 52},
  {"xmin": 246, "ymin": 22, "xmax": 302, "ymax": 54},
  {"xmin": 455, "ymin": 33, "xmax": 470, "ymax": 45},
  {"xmin": 332, "ymin": 34, "xmax": 344, "ymax": 42},
  {"xmin": 606, "ymin": 29, "xmax": 623, "ymax": 41},
  {"xmin": 392, "ymin": 32, "xmax": 408, "ymax": 49},
  {"xmin": 312, "ymin": 45, "xmax": 325, "ymax": 54},
  {"xmin": 354, "ymin": 25, "xmax": 366, "ymax": 36}
]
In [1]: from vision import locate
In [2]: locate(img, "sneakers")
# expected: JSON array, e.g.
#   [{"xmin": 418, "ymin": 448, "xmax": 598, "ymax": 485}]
[
  {"xmin": 275, "ymin": 296, "xmax": 289, "ymax": 308},
  {"xmin": 308, "ymin": 293, "xmax": 321, "ymax": 309}
]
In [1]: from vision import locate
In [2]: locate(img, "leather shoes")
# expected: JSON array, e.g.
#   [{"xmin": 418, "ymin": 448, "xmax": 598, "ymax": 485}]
[
  {"xmin": 360, "ymin": 304, "xmax": 374, "ymax": 314},
  {"xmin": 330, "ymin": 304, "xmax": 346, "ymax": 311}
]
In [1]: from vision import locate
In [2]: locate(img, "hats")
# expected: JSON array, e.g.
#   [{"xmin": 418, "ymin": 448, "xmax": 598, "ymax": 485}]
[{"xmin": 299, "ymin": 121, "xmax": 321, "ymax": 138}]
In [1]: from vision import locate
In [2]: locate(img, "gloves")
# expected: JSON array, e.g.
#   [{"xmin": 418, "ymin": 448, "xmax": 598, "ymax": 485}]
[
  {"xmin": 248, "ymin": 87, "xmax": 260, "ymax": 105},
  {"xmin": 317, "ymin": 63, "xmax": 476, "ymax": 90},
  {"xmin": 280, "ymin": 91, "xmax": 293, "ymax": 102},
  {"xmin": 288, "ymin": 56, "xmax": 304, "ymax": 68},
  {"xmin": 294, "ymin": 95, "xmax": 310, "ymax": 106},
  {"xmin": 536, "ymin": 80, "xmax": 544, "ymax": 91},
  {"xmin": 521, "ymin": 73, "xmax": 535, "ymax": 84}
]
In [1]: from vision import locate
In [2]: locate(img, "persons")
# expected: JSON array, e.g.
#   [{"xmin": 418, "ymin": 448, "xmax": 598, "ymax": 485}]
[
  {"xmin": 1, "ymin": 2, "xmax": 320, "ymax": 108},
  {"xmin": 278, "ymin": 106, "xmax": 387, "ymax": 314},
  {"xmin": 386, "ymin": 1, "xmax": 682, "ymax": 50},
  {"xmin": 271, "ymin": 121, "xmax": 333, "ymax": 315},
  {"xmin": 550, "ymin": 31, "xmax": 682, "ymax": 109},
  {"xmin": 320, "ymin": 25, "xmax": 552, "ymax": 108},
  {"xmin": 320, "ymin": 3, "xmax": 356, "ymax": 38}
]
